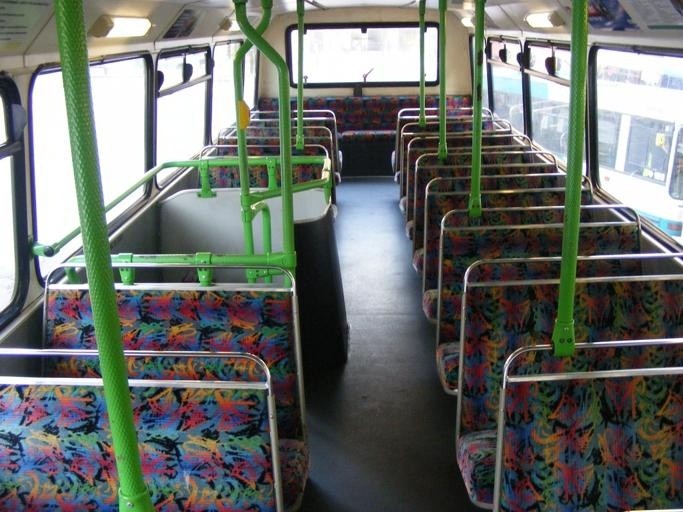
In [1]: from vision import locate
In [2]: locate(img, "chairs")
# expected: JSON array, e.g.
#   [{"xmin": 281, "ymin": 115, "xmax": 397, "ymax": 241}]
[
  {"xmin": 2, "ymin": 256, "xmax": 314, "ymax": 512},
  {"xmin": 346, "ymin": 96, "xmax": 682, "ymax": 506},
  {"xmin": 175, "ymin": 96, "xmax": 342, "ymax": 242}
]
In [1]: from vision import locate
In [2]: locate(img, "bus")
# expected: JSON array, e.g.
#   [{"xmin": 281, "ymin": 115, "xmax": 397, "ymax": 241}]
[{"xmin": 482, "ymin": 39, "xmax": 683, "ymax": 236}]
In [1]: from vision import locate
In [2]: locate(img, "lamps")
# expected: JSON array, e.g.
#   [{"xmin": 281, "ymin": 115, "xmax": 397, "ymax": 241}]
[
  {"xmin": 461, "ymin": 16, "xmax": 475, "ymax": 27},
  {"xmin": 526, "ymin": 11, "xmax": 564, "ymax": 29},
  {"xmin": 222, "ymin": 20, "xmax": 240, "ymax": 31},
  {"xmin": 90, "ymin": 15, "xmax": 151, "ymax": 38}
]
